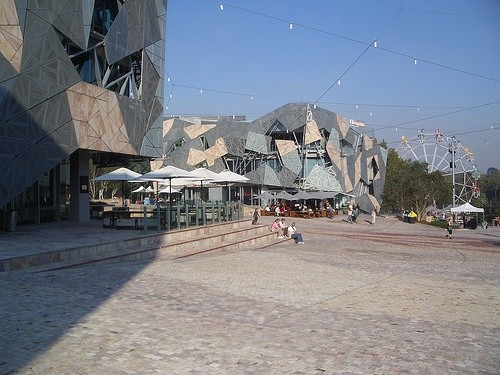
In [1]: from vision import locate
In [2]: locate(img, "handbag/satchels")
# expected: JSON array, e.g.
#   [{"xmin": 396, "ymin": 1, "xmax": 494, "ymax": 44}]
[{"xmin": 448, "ymin": 227, "xmax": 452, "ymax": 230}]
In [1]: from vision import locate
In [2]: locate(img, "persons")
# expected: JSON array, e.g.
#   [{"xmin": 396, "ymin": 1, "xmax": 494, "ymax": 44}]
[
  {"xmin": 252, "ymin": 208, "xmax": 260, "ymax": 222},
  {"xmin": 279, "ymin": 219, "xmax": 289, "ymax": 239},
  {"xmin": 287, "ymin": 222, "xmax": 304, "ymax": 244},
  {"xmin": 445, "ymin": 218, "xmax": 453, "ymax": 239},
  {"xmin": 370, "ymin": 211, "xmax": 376, "ymax": 224},
  {"xmin": 296, "ymin": 203, "xmax": 334, "ymax": 219},
  {"xmin": 271, "ymin": 219, "xmax": 283, "ymax": 238},
  {"xmin": 347, "ymin": 203, "xmax": 361, "ymax": 224},
  {"xmin": 265, "ymin": 203, "xmax": 291, "ymax": 216}
]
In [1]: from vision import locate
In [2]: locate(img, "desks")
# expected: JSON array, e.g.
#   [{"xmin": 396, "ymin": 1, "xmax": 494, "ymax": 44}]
[{"xmin": 289, "ymin": 211, "xmax": 314, "ymax": 218}]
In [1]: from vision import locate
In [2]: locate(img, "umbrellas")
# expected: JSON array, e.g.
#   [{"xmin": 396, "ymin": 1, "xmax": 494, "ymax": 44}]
[
  {"xmin": 89, "ymin": 167, "xmax": 142, "ymax": 207},
  {"xmin": 127, "ymin": 165, "xmax": 251, "ymax": 227},
  {"xmin": 249, "ymin": 190, "xmax": 339, "ymax": 200}
]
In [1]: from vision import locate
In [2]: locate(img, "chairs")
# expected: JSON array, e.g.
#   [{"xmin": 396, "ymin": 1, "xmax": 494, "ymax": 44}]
[{"xmin": 262, "ymin": 207, "xmax": 334, "ymax": 218}]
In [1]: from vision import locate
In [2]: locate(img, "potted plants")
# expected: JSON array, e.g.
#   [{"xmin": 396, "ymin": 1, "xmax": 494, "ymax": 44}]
[{"xmin": 403, "ymin": 210, "xmax": 417, "ymax": 223}]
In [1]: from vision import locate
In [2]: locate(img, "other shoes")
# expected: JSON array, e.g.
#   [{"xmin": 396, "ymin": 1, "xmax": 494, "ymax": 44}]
[
  {"xmin": 278, "ymin": 236, "xmax": 285, "ymax": 238},
  {"xmin": 450, "ymin": 237, "xmax": 452, "ymax": 239},
  {"xmin": 446, "ymin": 235, "xmax": 448, "ymax": 239}
]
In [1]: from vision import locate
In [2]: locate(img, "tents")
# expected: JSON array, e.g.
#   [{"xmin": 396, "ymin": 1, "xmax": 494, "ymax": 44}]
[{"xmin": 451, "ymin": 202, "xmax": 484, "ymax": 228}]
